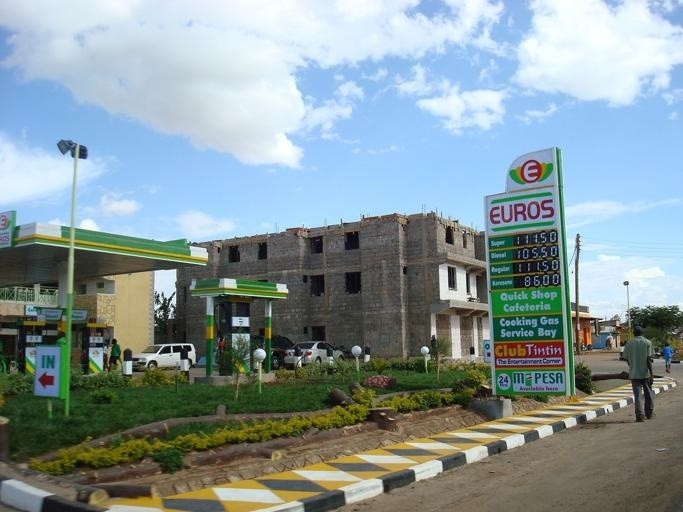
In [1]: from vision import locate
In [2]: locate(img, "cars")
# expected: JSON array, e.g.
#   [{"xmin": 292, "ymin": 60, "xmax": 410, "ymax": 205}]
[{"xmin": 285, "ymin": 341, "xmax": 346, "ymax": 366}]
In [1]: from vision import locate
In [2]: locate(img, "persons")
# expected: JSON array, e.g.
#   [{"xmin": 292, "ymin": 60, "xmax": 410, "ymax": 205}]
[
  {"xmin": 622, "ymin": 325, "xmax": 655, "ymax": 422},
  {"xmin": 55, "ymin": 331, "xmax": 65, "ymax": 346},
  {"xmin": 108, "ymin": 338, "xmax": 122, "ymax": 372},
  {"xmin": 662, "ymin": 342, "xmax": 673, "ymax": 373},
  {"xmin": 103, "ymin": 339, "xmax": 108, "ymax": 370}
]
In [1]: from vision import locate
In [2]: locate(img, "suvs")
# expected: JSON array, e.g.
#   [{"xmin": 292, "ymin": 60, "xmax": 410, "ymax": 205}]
[{"xmin": 249, "ymin": 334, "xmax": 297, "ymax": 370}]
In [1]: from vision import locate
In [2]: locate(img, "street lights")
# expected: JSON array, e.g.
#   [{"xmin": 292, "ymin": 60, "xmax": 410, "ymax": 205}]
[
  {"xmin": 621, "ymin": 280, "xmax": 631, "ymax": 338},
  {"xmin": 54, "ymin": 136, "xmax": 87, "ymax": 419}
]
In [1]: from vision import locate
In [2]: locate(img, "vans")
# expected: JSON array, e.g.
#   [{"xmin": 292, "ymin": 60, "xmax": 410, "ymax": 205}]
[{"xmin": 132, "ymin": 341, "xmax": 197, "ymax": 370}]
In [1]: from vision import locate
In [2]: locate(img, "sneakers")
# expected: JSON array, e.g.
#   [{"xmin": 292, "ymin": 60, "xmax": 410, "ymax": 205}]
[
  {"xmin": 636, "ymin": 416, "xmax": 647, "ymax": 422},
  {"xmin": 646, "ymin": 413, "xmax": 656, "ymax": 419}
]
